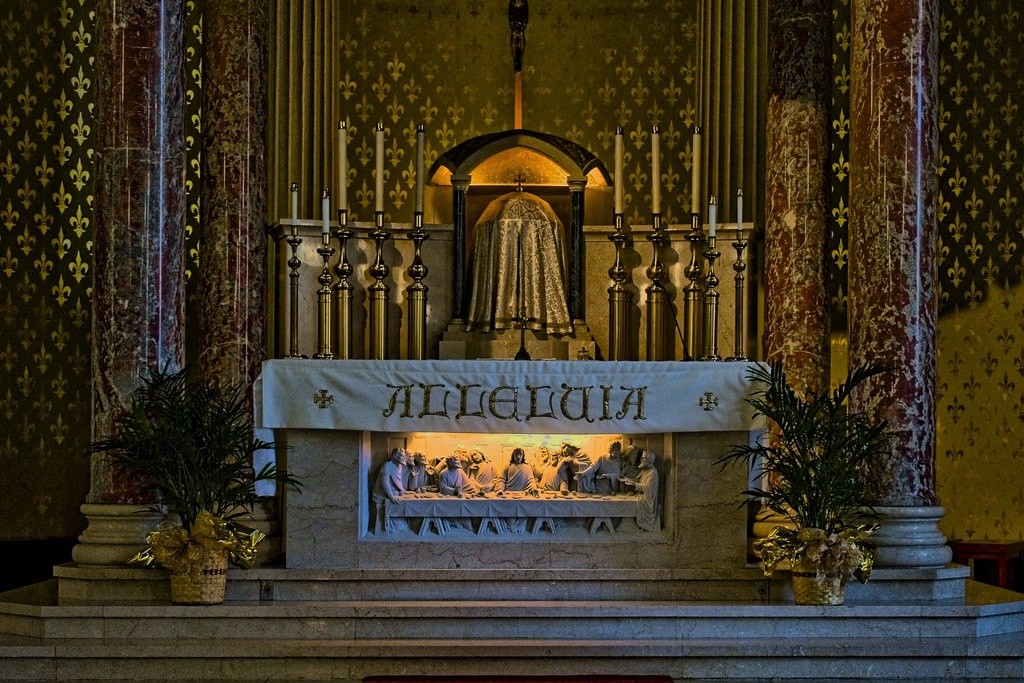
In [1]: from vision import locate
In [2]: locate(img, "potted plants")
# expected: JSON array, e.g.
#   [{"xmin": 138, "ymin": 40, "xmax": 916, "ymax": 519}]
[
  {"xmin": 77, "ymin": 350, "xmax": 304, "ymax": 603},
  {"xmin": 705, "ymin": 350, "xmax": 905, "ymax": 607}
]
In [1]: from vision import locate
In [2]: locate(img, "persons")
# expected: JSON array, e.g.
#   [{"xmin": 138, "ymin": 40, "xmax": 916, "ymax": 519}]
[
  {"xmin": 495, "ymin": 448, "xmax": 541, "ymax": 499},
  {"xmin": 372, "ymin": 440, "xmax": 661, "ymax": 534}
]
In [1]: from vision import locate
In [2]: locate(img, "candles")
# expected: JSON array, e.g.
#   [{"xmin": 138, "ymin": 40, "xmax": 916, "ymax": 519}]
[
  {"xmin": 416, "ymin": 124, "xmax": 424, "ymax": 212},
  {"xmin": 736, "ymin": 188, "xmax": 743, "ymax": 231},
  {"xmin": 708, "ymin": 195, "xmax": 716, "ymax": 236},
  {"xmin": 338, "ymin": 120, "xmax": 346, "ymax": 210},
  {"xmin": 692, "ymin": 125, "xmax": 700, "ymax": 213},
  {"xmin": 651, "ymin": 125, "xmax": 661, "ymax": 214},
  {"xmin": 322, "ymin": 188, "xmax": 329, "ymax": 234},
  {"xmin": 290, "ymin": 183, "xmax": 298, "ymax": 219},
  {"xmin": 376, "ymin": 122, "xmax": 384, "ymax": 211},
  {"xmin": 615, "ymin": 126, "xmax": 625, "ymax": 214}
]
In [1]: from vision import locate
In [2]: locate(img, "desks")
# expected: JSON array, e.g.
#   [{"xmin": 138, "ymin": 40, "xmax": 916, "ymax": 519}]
[{"xmin": 954, "ymin": 538, "xmax": 1024, "ymax": 593}]
